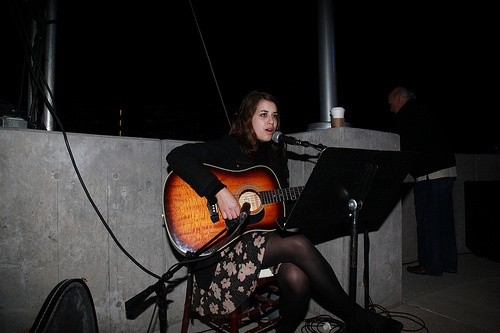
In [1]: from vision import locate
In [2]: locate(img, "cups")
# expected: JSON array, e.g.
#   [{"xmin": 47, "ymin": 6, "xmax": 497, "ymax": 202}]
[{"xmin": 331, "ymin": 107, "xmax": 345, "ymax": 128}]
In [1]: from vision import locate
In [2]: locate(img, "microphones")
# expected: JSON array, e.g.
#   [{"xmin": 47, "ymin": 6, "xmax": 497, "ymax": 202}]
[
  {"xmin": 273, "ymin": 131, "xmax": 309, "ymax": 147},
  {"xmin": 240, "ymin": 202, "xmax": 250, "ymax": 223}
]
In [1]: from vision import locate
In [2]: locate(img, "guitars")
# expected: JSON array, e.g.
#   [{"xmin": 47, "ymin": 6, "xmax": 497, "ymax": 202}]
[{"xmin": 161, "ymin": 163, "xmax": 306, "ymax": 262}]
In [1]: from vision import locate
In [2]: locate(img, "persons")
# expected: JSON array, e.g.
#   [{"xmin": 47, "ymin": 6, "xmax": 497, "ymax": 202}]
[
  {"xmin": 388, "ymin": 87, "xmax": 458, "ymax": 276},
  {"xmin": 167, "ymin": 91, "xmax": 403, "ymax": 333}
]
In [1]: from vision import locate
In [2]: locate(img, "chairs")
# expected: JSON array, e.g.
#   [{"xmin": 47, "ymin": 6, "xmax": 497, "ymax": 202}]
[{"xmin": 181, "ymin": 259, "xmax": 283, "ymax": 333}]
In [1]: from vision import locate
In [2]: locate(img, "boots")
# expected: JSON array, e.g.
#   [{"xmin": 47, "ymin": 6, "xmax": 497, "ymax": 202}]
[
  {"xmin": 312, "ymin": 270, "xmax": 404, "ymax": 333},
  {"xmin": 276, "ymin": 290, "xmax": 310, "ymax": 333}
]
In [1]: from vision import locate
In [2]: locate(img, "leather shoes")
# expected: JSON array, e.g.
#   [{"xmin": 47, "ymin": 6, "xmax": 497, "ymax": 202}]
[{"xmin": 406, "ymin": 264, "xmax": 443, "ymax": 276}]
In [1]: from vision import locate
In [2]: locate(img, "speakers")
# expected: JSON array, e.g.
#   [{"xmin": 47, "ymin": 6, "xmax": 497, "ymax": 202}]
[{"xmin": 464, "ymin": 180, "xmax": 500, "ymax": 263}]
